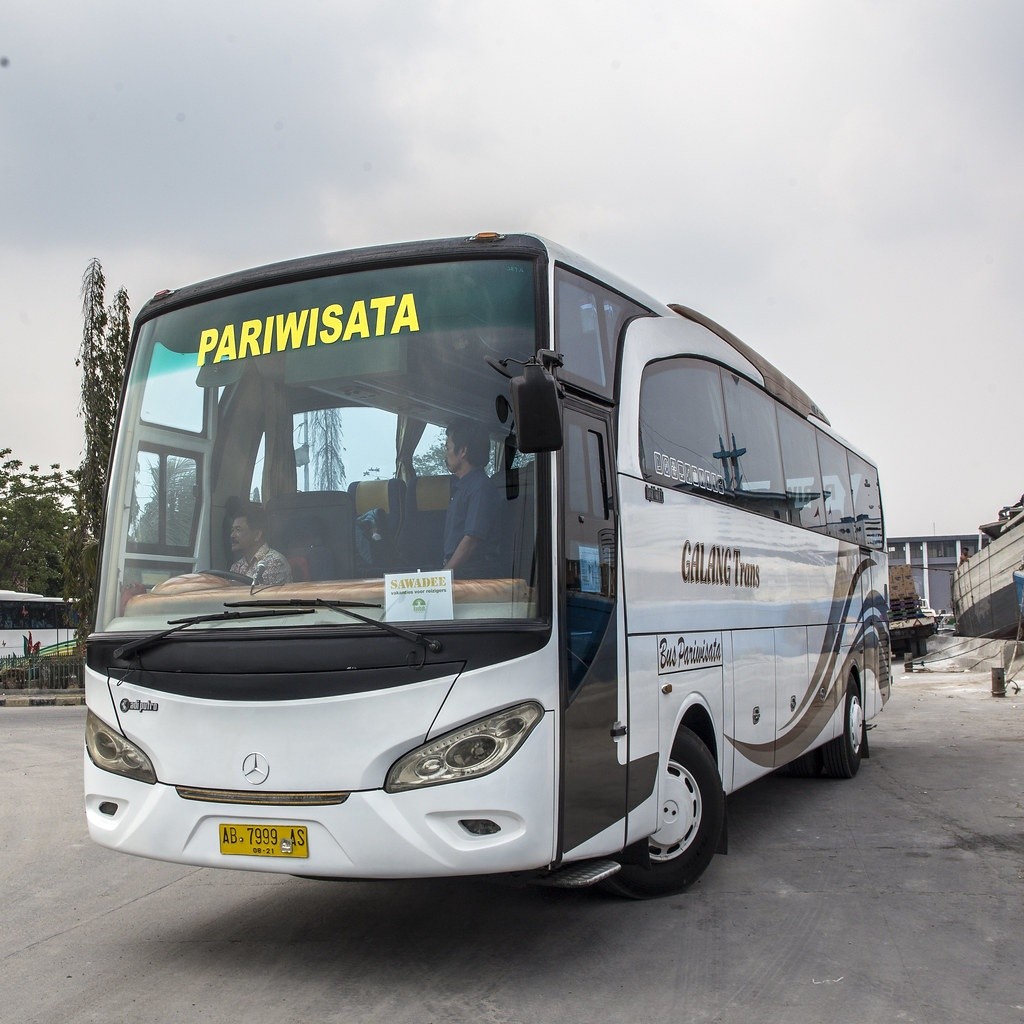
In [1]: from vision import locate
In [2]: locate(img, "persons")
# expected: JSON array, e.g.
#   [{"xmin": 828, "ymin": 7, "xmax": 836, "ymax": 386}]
[
  {"xmin": 439, "ymin": 418, "xmax": 508, "ymax": 579},
  {"xmin": 228, "ymin": 506, "xmax": 293, "ymax": 586},
  {"xmin": 959, "ymin": 547, "xmax": 970, "ymax": 566}
]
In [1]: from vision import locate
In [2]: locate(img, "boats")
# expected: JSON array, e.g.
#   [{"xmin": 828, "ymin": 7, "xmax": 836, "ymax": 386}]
[{"xmin": 950, "ymin": 523, "xmax": 1024, "ymax": 640}]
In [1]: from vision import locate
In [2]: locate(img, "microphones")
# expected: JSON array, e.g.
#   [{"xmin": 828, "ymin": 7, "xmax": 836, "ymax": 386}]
[{"xmin": 250, "ymin": 561, "xmax": 267, "ymax": 594}]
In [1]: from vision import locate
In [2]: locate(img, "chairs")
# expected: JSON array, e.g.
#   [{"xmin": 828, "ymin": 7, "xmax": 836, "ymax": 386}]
[
  {"xmin": 264, "ymin": 491, "xmax": 355, "ymax": 582},
  {"xmin": 346, "ymin": 472, "xmax": 461, "ymax": 576}
]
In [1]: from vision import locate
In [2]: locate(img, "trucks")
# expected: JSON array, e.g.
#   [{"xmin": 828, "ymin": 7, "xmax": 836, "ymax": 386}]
[{"xmin": 888, "ymin": 598, "xmax": 936, "ymax": 658}]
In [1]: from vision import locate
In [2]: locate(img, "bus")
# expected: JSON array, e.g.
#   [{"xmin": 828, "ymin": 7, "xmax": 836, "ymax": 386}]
[
  {"xmin": 82, "ymin": 222, "xmax": 893, "ymax": 902},
  {"xmin": 0, "ymin": 589, "xmax": 89, "ymax": 689}
]
